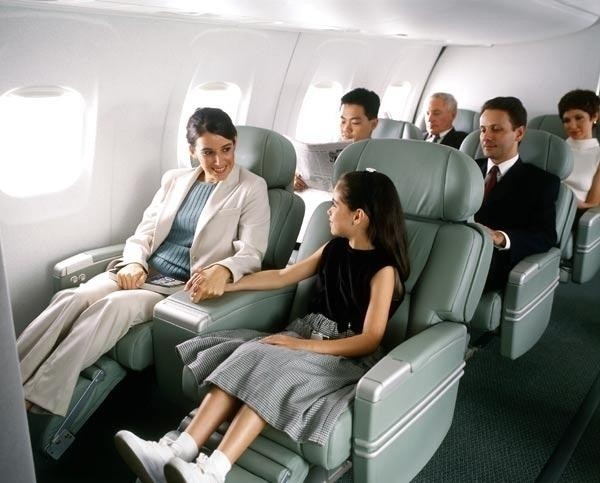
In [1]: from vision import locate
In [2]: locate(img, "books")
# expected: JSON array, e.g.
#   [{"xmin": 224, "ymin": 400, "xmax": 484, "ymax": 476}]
[
  {"xmin": 279, "ymin": 134, "xmax": 355, "ymax": 193},
  {"xmin": 107, "ymin": 266, "xmax": 187, "ymax": 296}
]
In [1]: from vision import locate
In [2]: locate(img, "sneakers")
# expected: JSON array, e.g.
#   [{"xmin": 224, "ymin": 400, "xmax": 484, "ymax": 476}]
[{"xmin": 113, "ymin": 429, "xmax": 225, "ymax": 483}]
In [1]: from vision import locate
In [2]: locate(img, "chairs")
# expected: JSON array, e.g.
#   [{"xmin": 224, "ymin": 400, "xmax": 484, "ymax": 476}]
[
  {"xmin": 371, "ymin": 116, "xmax": 424, "ymax": 142},
  {"xmin": 136, "ymin": 138, "xmax": 494, "ymax": 483},
  {"xmin": 528, "ymin": 115, "xmax": 599, "ymax": 284},
  {"xmin": 27, "ymin": 125, "xmax": 305, "ymax": 461},
  {"xmin": 421, "ymin": 109, "xmax": 481, "ymax": 134},
  {"xmin": 459, "ymin": 128, "xmax": 574, "ymax": 359}
]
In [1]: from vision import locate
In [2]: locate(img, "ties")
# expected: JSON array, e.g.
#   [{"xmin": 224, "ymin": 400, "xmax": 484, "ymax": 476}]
[
  {"xmin": 432, "ymin": 133, "xmax": 440, "ymax": 143},
  {"xmin": 483, "ymin": 165, "xmax": 499, "ymax": 201}
]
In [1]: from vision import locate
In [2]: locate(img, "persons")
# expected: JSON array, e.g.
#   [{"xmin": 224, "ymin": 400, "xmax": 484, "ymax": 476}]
[
  {"xmin": 16, "ymin": 106, "xmax": 272, "ymax": 417},
  {"xmin": 292, "ymin": 87, "xmax": 381, "ymax": 192},
  {"xmin": 557, "ymin": 89, "xmax": 600, "ymax": 209},
  {"xmin": 422, "ymin": 92, "xmax": 469, "ymax": 149},
  {"xmin": 113, "ymin": 167, "xmax": 411, "ymax": 482},
  {"xmin": 473, "ymin": 96, "xmax": 560, "ymax": 292}
]
